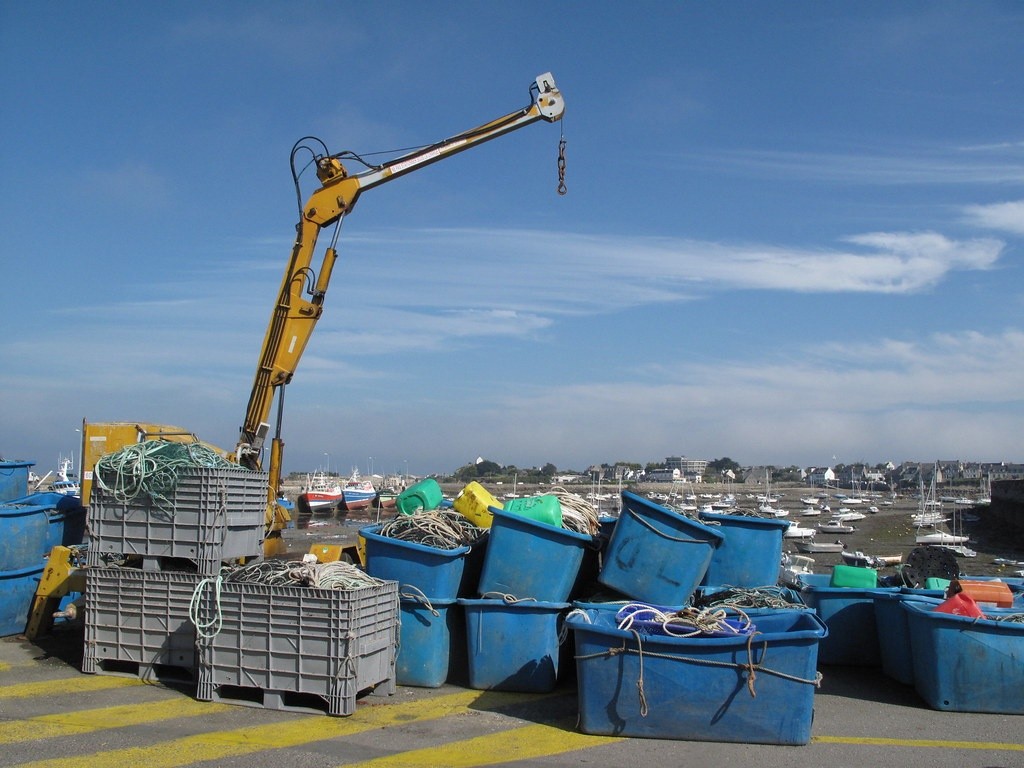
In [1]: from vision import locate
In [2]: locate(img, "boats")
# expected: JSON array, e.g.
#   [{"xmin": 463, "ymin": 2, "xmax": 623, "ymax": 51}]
[
  {"xmin": 574, "ymin": 470, "xmax": 622, "ymax": 517},
  {"xmin": 841, "ymin": 551, "xmax": 903, "ymax": 567},
  {"xmin": 784, "ymin": 521, "xmax": 816, "ymax": 537},
  {"xmin": 780, "ymin": 550, "xmax": 815, "ymax": 589},
  {"xmin": 647, "ymin": 469, "xmax": 790, "ymax": 517},
  {"xmin": 372, "ymin": 488, "xmax": 399, "ymax": 508},
  {"xmin": 831, "ymin": 507, "xmax": 867, "ymax": 522},
  {"xmin": 817, "ymin": 517, "xmax": 855, "ymax": 534},
  {"xmin": 492, "ymin": 491, "xmax": 544, "ymax": 501},
  {"xmin": 341, "ymin": 465, "xmax": 377, "ymax": 511},
  {"xmin": 799, "ymin": 506, "xmax": 821, "ymax": 516},
  {"xmin": 868, "ymin": 507, "xmax": 879, "ymax": 513},
  {"xmin": 298, "ymin": 465, "xmax": 343, "ymax": 512},
  {"xmin": 793, "ymin": 531, "xmax": 848, "ymax": 554},
  {"xmin": 993, "ymin": 558, "xmax": 1024, "ymax": 577}
]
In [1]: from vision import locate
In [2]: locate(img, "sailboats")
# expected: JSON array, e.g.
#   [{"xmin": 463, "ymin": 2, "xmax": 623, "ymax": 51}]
[
  {"xmin": 913, "ymin": 462, "xmax": 991, "ymax": 558},
  {"xmin": 800, "ymin": 469, "xmax": 898, "ymax": 510}
]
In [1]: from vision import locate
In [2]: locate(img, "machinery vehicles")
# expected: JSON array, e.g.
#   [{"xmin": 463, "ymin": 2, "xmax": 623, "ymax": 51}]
[{"xmin": 19, "ymin": 72, "xmax": 566, "ymax": 640}]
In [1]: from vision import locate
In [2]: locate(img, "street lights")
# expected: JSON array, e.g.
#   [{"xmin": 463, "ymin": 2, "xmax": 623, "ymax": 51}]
[
  {"xmin": 369, "ymin": 456, "xmax": 373, "ymax": 481},
  {"xmin": 404, "ymin": 459, "xmax": 408, "ymax": 486},
  {"xmin": 324, "ymin": 453, "xmax": 329, "ymax": 482}
]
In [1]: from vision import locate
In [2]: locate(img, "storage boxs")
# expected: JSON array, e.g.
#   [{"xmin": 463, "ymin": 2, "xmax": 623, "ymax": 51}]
[
  {"xmin": 82, "ymin": 564, "xmax": 243, "ymax": 679},
  {"xmin": 359, "ymin": 520, "xmax": 489, "ymax": 599},
  {"xmin": 565, "ymin": 605, "xmax": 829, "ymax": 745},
  {"xmin": 478, "ymin": 504, "xmax": 594, "ymax": 603},
  {"xmin": 197, "ymin": 575, "xmax": 399, "ymax": 717},
  {"xmin": 572, "ymin": 586, "xmax": 817, "ymax": 615},
  {"xmin": 573, "ymin": 517, "xmax": 618, "ymax": 597},
  {"xmin": 871, "ymin": 576, "xmax": 1024, "ymax": 695},
  {"xmin": 458, "ymin": 598, "xmax": 579, "ymax": 693},
  {"xmin": 598, "ymin": 491, "xmax": 726, "ymax": 608},
  {"xmin": 396, "ymin": 599, "xmax": 463, "ymax": 688},
  {"xmin": 899, "ymin": 600, "xmax": 1024, "ymax": 715},
  {"xmin": 698, "ymin": 512, "xmax": 790, "ymax": 589},
  {"xmin": 85, "ymin": 465, "xmax": 269, "ymax": 577},
  {"xmin": 0, "ymin": 458, "xmax": 86, "ymax": 638},
  {"xmin": 796, "ymin": 574, "xmax": 902, "ymax": 666}
]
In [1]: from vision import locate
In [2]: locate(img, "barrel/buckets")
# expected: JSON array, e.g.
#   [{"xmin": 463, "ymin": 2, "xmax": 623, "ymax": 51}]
[
  {"xmin": 453, "ymin": 481, "xmax": 503, "ymax": 528},
  {"xmin": 831, "ymin": 565, "xmax": 877, "ymax": 588},
  {"xmin": 503, "ymin": 495, "xmax": 562, "ymax": 528},
  {"xmin": 925, "ymin": 577, "xmax": 951, "ymax": 590},
  {"xmin": 396, "ymin": 478, "xmax": 443, "ymax": 516}
]
